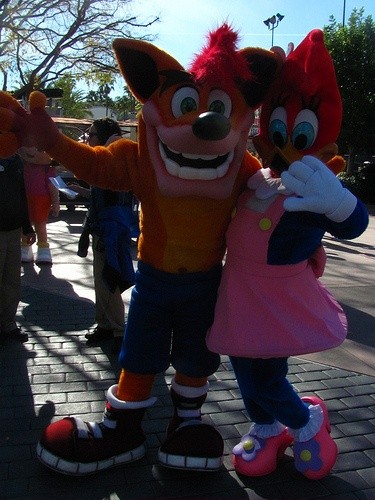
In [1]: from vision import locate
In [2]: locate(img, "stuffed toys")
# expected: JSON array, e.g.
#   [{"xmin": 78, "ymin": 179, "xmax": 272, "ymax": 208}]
[
  {"xmin": 205, "ymin": 30, "xmax": 369, "ymax": 480},
  {"xmin": 0, "ymin": 25, "xmax": 346, "ymax": 479},
  {"xmin": 17, "ymin": 146, "xmax": 60, "ymax": 265}
]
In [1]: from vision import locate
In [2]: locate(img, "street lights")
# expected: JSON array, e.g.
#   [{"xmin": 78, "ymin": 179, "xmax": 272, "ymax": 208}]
[{"xmin": 262, "ymin": 14, "xmax": 285, "ymax": 47}]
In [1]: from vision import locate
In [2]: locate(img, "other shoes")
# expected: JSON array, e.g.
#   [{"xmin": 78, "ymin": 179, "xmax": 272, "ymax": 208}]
[
  {"xmin": 0, "ymin": 328, "xmax": 28, "ymax": 341},
  {"xmin": 85, "ymin": 326, "xmax": 123, "ymax": 348}
]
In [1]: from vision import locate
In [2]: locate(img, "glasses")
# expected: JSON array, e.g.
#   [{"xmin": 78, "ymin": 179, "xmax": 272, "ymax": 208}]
[{"xmin": 87, "ymin": 131, "xmax": 98, "ymax": 137}]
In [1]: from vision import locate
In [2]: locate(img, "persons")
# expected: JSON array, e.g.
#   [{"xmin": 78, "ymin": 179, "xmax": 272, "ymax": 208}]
[
  {"xmin": 77, "ymin": 118, "xmax": 139, "ymax": 350},
  {"xmin": 0, "ymin": 154, "xmax": 36, "ymax": 348}
]
